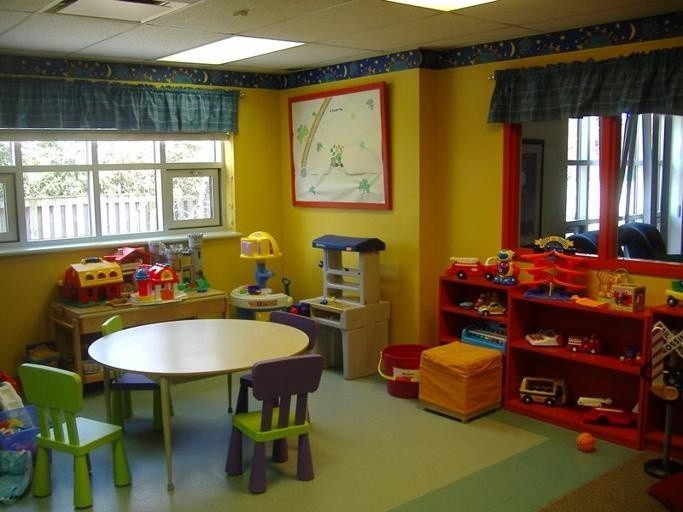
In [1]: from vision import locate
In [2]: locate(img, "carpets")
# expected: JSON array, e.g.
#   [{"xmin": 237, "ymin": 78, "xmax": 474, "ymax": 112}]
[{"xmin": 534, "ymin": 448, "xmax": 682, "ymax": 512}]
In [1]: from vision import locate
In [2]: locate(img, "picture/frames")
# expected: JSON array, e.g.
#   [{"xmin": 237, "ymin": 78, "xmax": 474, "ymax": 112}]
[
  {"xmin": 520, "ymin": 137, "xmax": 546, "ymax": 246},
  {"xmin": 284, "ymin": 79, "xmax": 396, "ymax": 212}
]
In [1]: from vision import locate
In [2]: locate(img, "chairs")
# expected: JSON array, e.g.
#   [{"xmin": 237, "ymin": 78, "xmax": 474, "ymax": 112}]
[
  {"xmin": 233, "ymin": 308, "xmax": 316, "ymax": 425},
  {"xmin": 97, "ymin": 313, "xmax": 174, "ymax": 435},
  {"xmin": 221, "ymin": 352, "xmax": 326, "ymax": 496},
  {"xmin": 17, "ymin": 361, "xmax": 138, "ymax": 511}
]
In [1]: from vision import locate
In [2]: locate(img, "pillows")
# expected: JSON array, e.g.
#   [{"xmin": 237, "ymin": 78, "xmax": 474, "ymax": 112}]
[{"xmin": 642, "ymin": 469, "xmax": 682, "ymax": 512}]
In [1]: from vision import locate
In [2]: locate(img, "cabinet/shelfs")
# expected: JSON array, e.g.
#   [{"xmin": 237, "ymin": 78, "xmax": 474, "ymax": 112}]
[
  {"xmin": 641, "ymin": 300, "xmax": 681, "ymax": 462},
  {"xmin": 433, "ymin": 269, "xmax": 532, "ymax": 411},
  {"xmin": 310, "ymin": 231, "xmax": 387, "ymax": 309},
  {"xmin": 499, "ymin": 281, "xmax": 650, "ymax": 454}
]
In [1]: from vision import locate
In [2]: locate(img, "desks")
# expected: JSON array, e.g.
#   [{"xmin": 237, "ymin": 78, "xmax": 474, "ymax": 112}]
[
  {"xmin": 39, "ymin": 285, "xmax": 230, "ymax": 399},
  {"xmin": 84, "ymin": 317, "xmax": 311, "ymax": 494},
  {"xmin": 297, "ymin": 292, "xmax": 393, "ymax": 384}
]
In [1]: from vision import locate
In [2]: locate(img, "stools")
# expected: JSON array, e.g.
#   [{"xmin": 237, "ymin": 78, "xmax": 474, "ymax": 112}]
[{"xmin": 414, "ymin": 338, "xmax": 504, "ymax": 427}]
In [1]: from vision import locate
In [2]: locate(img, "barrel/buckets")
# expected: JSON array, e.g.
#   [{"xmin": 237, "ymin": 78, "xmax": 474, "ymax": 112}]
[{"xmin": 376, "ymin": 343, "xmax": 435, "ymax": 400}]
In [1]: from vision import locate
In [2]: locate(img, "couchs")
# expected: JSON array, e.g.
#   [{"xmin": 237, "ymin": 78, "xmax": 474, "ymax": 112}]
[{"xmin": 562, "ymin": 218, "xmax": 681, "ymax": 262}]
